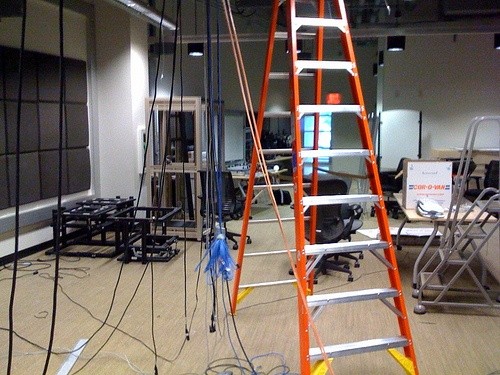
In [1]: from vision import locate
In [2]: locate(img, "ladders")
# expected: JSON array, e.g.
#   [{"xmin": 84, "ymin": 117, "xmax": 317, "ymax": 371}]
[
  {"xmin": 229, "ymin": 0, "xmax": 418, "ymax": 375},
  {"xmin": 410, "ymin": 114, "xmax": 499, "ymax": 314}
]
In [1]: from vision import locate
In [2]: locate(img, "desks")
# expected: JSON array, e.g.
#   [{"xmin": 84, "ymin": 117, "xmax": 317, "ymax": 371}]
[
  {"xmin": 230, "ymin": 167, "xmax": 288, "ymax": 199},
  {"xmin": 392, "ymin": 191, "xmax": 498, "ymax": 294}
]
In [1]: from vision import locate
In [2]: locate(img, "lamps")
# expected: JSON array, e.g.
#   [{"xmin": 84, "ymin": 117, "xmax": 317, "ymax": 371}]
[
  {"xmin": 187, "ymin": 43, "xmax": 204, "ymax": 57},
  {"xmin": 372, "ymin": 63, "xmax": 377, "ymax": 77},
  {"xmin": 379, "ymin": 51, "xmax": 384, "ymax": 67},
  {"xmin": 493, "ymin": 33, "xmax": 500, "ymax": 50},
  {"xmin": 283, "ymin": 38, "xmax": 303, "ymax": 54},
  {"xmin": 386, "ymin": 36, "xmax": 406, "ymax": 52}
]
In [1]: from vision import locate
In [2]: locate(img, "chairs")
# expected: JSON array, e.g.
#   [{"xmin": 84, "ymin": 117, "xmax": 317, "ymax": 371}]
[
  {"xmin": 465, "ymin": 158, "xmax": 500, "ymax": 201},
  {"xmin": 445, "ymin": 156, "xmax": 477, "ymax": 186},
  {"xmin": 299, "ymin": 177, "xmax": 368, "ymax": 285},
  {"xmin": 369, "ymin": 155, "xmax": 410, "ymax": 219}
]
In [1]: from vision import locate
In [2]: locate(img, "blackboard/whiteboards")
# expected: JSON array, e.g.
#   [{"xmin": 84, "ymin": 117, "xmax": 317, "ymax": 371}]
[{"xmin": 378, "ymin": 109, "xmax": 421, "ymax": 169}]
